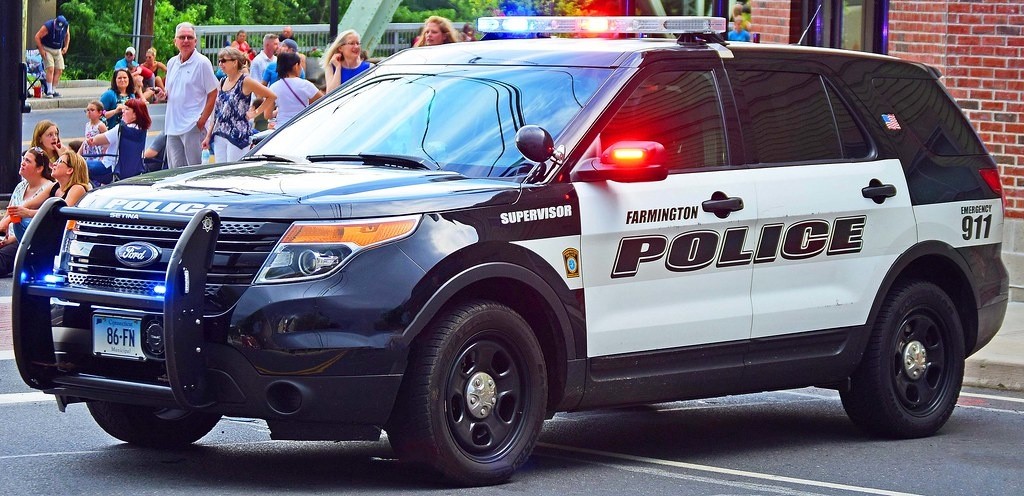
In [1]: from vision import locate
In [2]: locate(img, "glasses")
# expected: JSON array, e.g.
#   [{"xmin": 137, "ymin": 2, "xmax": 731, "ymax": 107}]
[
  {"xmin": 341, "ymin": 41, "xmax": 362, "ymax": 46},
  {"xmin": 176, "ymin": 35, "xmax": 194, "ymax": 40},
  {"xmin": 56, "ymin": 158, "xmax": 69, "ymax": 167},
  {"xmin": 219, "ymin": 58, "xmax": 235, "ymax": 63},
  {"xmin": 31, "ymin": 146, "xmax": 45, "ymax": 166}
]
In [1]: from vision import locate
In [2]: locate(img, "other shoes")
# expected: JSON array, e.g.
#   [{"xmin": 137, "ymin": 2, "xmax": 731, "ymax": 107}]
[
  {"xmin": 53, "ymin": 92, "xmax": 63, "ymax": 98},
  {"xmin": 47, "ymin": 93, "xmax": 53, "ymax": 98}
]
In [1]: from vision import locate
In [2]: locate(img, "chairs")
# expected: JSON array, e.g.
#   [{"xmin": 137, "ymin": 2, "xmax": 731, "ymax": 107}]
[
  {"xmin": 23, "ymin": 61, "xmax": 47, "ymax": 95},
  {"xmin": 80, "ymin": 118, "xmax": 169, "ymax": 188},
  {"xmin": 634, "ymin": 90, "xmax": 703, "ymax": 168}
]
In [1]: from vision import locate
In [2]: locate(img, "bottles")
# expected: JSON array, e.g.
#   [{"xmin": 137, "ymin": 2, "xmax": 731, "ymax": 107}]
[{"xmin": 201, "ymin": 146, "xmax": 210, "ymax": 164}]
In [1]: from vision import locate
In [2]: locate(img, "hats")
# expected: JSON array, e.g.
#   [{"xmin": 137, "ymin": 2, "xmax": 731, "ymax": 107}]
[
  {"xmin": 126, "ymin": 47, "xmax": 135, "ymax": 54},
  {"xmin": 55, "ymin": 16, "xmax": 66, "ymax": 30},
  {"xmin": 279, "ymin": 39, "xmax": 298, "ymax": 52}
]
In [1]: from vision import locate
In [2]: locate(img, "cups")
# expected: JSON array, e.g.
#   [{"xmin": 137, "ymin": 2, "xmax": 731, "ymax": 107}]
[{"xmin": 6, "ymin": 206, "xmax": 21, "ymax": 223}]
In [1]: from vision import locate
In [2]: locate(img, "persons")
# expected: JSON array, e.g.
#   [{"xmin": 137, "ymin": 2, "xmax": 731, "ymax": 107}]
[
  {"xmin": 268, "ymin": 53, "xmax": 325, "ymax": 131},
  {"xmin": 262, "ymin": 38, "xmax": 305, "ymax": 87},
  {"xmin": 165, "ymin": 22, "xmax": 220, "ymax": 169},
  {"xmin": 100, "ymin": 69, "xmax": 135, "ymax": 131},
  {"xmin": 0, "ymin": 146, "xmax": 56, "ymax": 276},
  {"xmin": 8, "ymin": 151, "xmax": 89, "ymax": 244},
  {"xmin": 142, "ymin": 132, "xmax": 167, "ymax": 173},
  {"xmin": 115, "ymin": 46, "xmax": 167, "ymax": 104},
  {"xmin": 232, "ymin": 30, "xmax": 257, "ymax": 60},
  {"xmin": 77, "ymin": 100, "xmax": 108, "ymax": 160},
  {"xmin": 85, "ymin": 98, "xmax": 152, "ymax": 187},
  {"xmin": 202, "ymin": 47, "xmax": 278, "ymax": 163},
  {"xmin": 323, "ymin": 30, "xmax": 375, "ymax": 94},
  {"xmin": 30, "ymin": 120, "xmax": 67, "ymax": 168},
  {"xmin": 278, "ymin": 26, "xmax": 296, "ymax": 43},
  {"xmin": 251, "ymin": 33, "xmax": 280, "ymax": 88},
  {"xmin": 728, "ymin": 3, "xmax": 751, "ymax": 42},
  {"xmin": 35, "ymin": 16, "xmax": 70, "ymax": 98},
  {"xmin": 413, "ymin": 16, "xmax": 477, "ymax": 47}
]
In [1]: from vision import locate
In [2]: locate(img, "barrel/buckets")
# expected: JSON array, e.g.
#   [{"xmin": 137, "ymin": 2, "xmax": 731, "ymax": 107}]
[{"xmin": 34, "ymin": 86, "xmax": 41, "ymax": 97}]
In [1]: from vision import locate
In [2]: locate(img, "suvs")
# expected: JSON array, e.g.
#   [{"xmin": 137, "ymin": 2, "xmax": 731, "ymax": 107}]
[{"xmin": 12, "ymin": 15, "xmax": 1010, "ymax": 488}]
